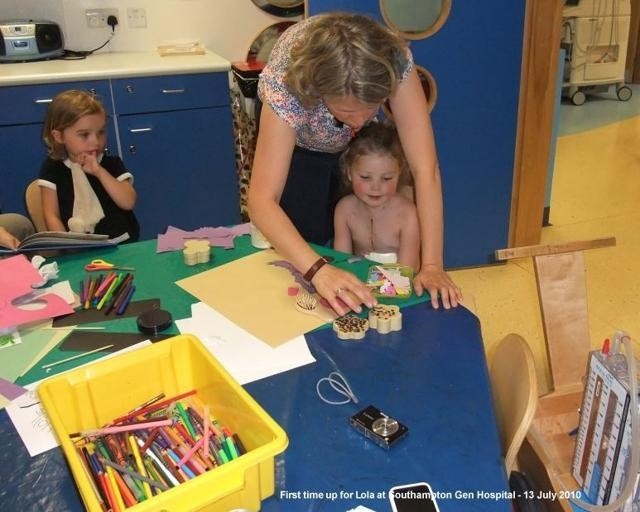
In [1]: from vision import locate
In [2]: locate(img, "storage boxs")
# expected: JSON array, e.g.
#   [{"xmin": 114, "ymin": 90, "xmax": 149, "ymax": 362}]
[{"xmin": 37, "ymin": 332, "xmax": 289, "ymax": 512}]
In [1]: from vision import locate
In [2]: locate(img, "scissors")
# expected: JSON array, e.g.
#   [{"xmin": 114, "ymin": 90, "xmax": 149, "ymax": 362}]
[{"xmin": 84, "ymin": 259, "xmax": 136, "ymax": 271}]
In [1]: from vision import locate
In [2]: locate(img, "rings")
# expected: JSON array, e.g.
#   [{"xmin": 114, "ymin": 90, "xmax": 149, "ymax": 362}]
[{"xmin": 336, "ymin": 287, "xmax": 347, "ymax": 294}]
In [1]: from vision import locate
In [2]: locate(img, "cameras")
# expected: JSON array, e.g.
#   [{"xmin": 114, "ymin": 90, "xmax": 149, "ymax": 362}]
[{"xmin": 350, "ymin": 404, "xmax": 409, "ymax": 449}]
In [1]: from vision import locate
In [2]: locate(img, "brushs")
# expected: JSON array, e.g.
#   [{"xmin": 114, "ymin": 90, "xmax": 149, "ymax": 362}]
[{"xmin": 294, "ymin": 292, "xmax": 335, "ymax": 322}]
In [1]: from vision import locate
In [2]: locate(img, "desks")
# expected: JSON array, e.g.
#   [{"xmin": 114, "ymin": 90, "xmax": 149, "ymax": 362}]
[{"xmin": 1, "ymin": 238, "xmax": 515, "ymax": 512}]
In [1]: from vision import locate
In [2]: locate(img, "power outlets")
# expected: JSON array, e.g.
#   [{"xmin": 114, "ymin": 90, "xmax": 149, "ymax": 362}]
[{"xmin": 85, "ymin": 7, "xmax": 118, "ymax": 27}]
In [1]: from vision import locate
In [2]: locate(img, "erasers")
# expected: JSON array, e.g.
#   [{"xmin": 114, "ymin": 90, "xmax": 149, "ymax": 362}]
[
  {"xmin": 182, "ymin": 240, "xmax": 210, "ymax": 266},
  {"xmin": 333, "ymin": 315, "xmax": 369, "ymax": 339},
  {"xmin": 368, "ymin": 305, "xmax": 402, "ymax": 334}
]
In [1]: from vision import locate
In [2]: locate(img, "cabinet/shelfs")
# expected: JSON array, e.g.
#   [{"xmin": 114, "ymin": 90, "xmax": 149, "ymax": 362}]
[
  {"xmin": 0, "ymin": 79, "xmax": 121, "ymax": 233},
  {"xmin": 110, "ymin": 70, "xmax": 244, "ymax": 240}
]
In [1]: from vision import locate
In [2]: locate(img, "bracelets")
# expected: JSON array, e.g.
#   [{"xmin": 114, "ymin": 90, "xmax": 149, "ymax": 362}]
[{"xmin": 302, "ymin": 253, "xmax": 331, "ymax": 278}]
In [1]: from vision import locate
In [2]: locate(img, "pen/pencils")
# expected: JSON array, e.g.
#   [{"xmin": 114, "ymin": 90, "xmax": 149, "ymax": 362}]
[
  {"xmin": 80, "ymin": 272, "xmax": 135, "ymax": 315},
  {"xmin": 69, "ymin": 390, "xmax": 247, "ymax": 512},
  {"xmin": 42, "ymin": 344, "xmax": 114, "ymax": 369}
]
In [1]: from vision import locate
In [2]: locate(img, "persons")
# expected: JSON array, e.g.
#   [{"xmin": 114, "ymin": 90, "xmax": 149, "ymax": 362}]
[
  {"xmin": 33, "ymin": 86, "xmax": 141, "ymax": 250},
  {"xmin": 328, "ymin": 122, "xmax": 422, "ymax": 281},
  {"xmin": 245, "ymin": 10, "xmax": 466, "ymax": 323}
]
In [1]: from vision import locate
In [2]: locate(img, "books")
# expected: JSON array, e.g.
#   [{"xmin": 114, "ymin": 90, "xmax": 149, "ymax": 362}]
[
  {"xmin": 155, "ymin": 38, "xmax": 207, "ymax": 58},
  {"xmin": 0, "ymin": 226, "xmax": 119, "ymax": 256}
]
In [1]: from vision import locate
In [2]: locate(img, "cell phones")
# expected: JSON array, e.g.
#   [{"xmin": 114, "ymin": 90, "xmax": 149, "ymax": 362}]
[{"xmin": 389, "ymin": 482, "xmax": 440, "ymax": 512}]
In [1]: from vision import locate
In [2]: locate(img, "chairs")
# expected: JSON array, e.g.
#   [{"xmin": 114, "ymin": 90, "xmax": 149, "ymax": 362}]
[
  {"xmin": 26, "ymin": 180, "xmax": 61, "ymax": 256},
  {"xmin": 488, "ymin": 334, "xmax": 540, "ymax": 479}
]
